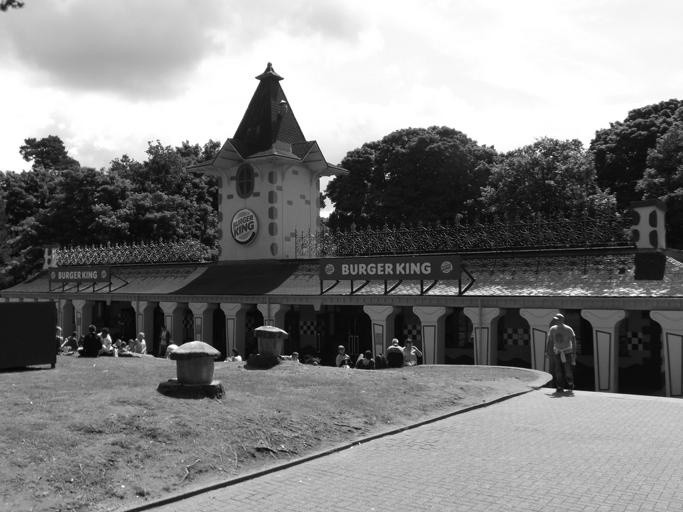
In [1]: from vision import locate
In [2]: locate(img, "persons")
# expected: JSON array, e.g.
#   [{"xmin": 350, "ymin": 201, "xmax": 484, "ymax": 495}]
[
  {"xmin": 164, "ymin": 339, "xmax": 179, "ymax": 359},
  {"xmin": 387, "ymin": 338, "xmax": 404, "ymax": 368},
  {"xmin": 287, "ymin": 352, "xmax": 299, "ymax": 362},
  {"xmin": 545, "ymin": 312, "xmax": 579, "ymax": 397},
  {"xmin": 232, "ymin": 352, "xmax": 242, "ymax": 362},
  {"xmin": 223, "ymin": 352, "xmax": 232, "ymax": 362},
  {"xmin": 231, "ymin": 348, "xmax": 237, "ymax": 361},
  {"xmin": 335, "ymin": 345, "xmax": 354, "ymax": 369},
  {"xmin": 355, "ymin": 350, "xmax": 375, "ymax": 369},
  {"xmin": 401, "ymin": 339, "xmax": 423, "ymax": 367},
  {"xmin": 56, "ymin": 324, "xmax": 153, "ymax": 359},
  {"xmin": 159, "ymin": 324, "xmax": 171, "ymax": 358}
]
